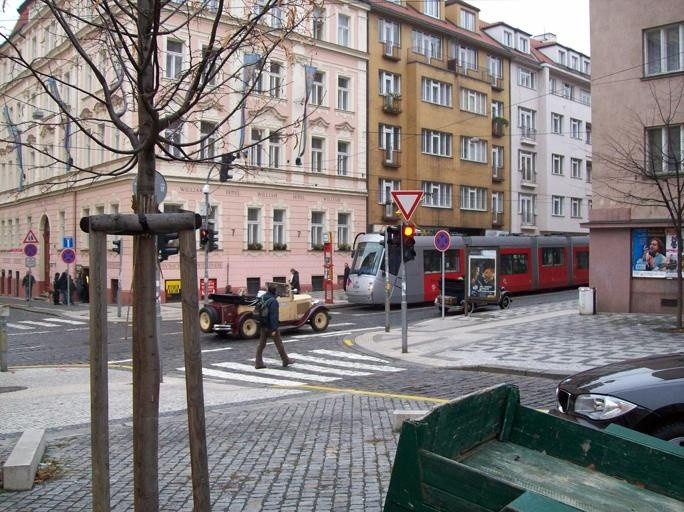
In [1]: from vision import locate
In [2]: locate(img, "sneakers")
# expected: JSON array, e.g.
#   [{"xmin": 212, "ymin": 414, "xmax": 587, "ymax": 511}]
[
  {"xmin": 254, "ymin": 364, "xmax": 267, "ymax": 370},
  {"xmin": 282, "ymin": 357, "xmax": 296, "ymax": 367}
]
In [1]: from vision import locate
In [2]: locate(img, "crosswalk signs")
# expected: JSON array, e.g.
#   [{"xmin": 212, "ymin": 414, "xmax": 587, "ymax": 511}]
[{"xmin": 23, "ymin": 230, "xmax": 39, "ymax": 243}]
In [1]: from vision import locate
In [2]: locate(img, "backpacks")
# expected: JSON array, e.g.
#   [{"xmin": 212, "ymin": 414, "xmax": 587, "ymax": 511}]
[{"xmin": 251, "ymin": 295, "xmax": 275, "ymax": 326}]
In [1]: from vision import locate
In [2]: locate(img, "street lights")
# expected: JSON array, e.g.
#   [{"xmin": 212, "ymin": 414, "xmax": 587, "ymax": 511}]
[{"xmin": 199, "ymin": 153, "xmax": 237, "ymax": 305}]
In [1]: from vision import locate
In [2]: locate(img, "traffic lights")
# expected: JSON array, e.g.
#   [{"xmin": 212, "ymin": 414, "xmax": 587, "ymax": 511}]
[
  {"xmin": 219, "ymin": 153, "xmax": 237, "ymax": 182},
  {"xmin": 112, "ymin": 240, "xmax": 120, "ymax": 254},
  {"xmin": 158, "ymin": 233, "xmax": 178, "ymax": 262},
  {"xmin": 380, "ymin": 224, "xmax": 416, "ymax": 261},
  {"xmin": 199, "ymin": 229, "xmax": 218, "ymax": 252}
]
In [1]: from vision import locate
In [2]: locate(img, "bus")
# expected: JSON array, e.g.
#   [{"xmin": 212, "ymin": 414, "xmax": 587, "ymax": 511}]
[{"xmin": 344, "ymin": 232, "xmax": 589, "ymax": 304}]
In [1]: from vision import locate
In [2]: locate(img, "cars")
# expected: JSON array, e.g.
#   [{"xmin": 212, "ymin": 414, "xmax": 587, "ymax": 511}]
[
  {"xmin": 434, "ymin": 277, "xmax": 512, "ymax": 312},
  {"xmin": 548, "ymin": 352, "xmax": 684, "ymax": 446},
  {"xmin": 199, "ymin": 282, "xmax": 332, "ymax": 339}
]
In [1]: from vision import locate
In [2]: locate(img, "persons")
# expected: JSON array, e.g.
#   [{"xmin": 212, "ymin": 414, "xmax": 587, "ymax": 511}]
[
  {"xmin": 254, "ymin": 285, "xmax": 295, "ymax": 369},
  {"xmin": 225, "ymin": 285, "xmax": 232, "ymax": 294},
  {"xmin": 470, "ymin": 262, "xmax": 481, "ymax": 285},
  {"xmin": 20, "ymin": 270, "xmax": 35, "ymax": 301},
  {"xmin": 642, "ymin": 237, "xmax": 666, "ymax": 270},
  {"xmin": 667, "ymin": 253, "xmax": 677, "ymax": 271},
  {"xmin": 671, "ymin": 230, "xmax": 678, "ymax": 249},
  {"xmin": 287, "ymin": 268, "xmax": 301, "ymax": 294},
  {"xmin": 53, "ymin": 271, "xmax": 86, "ymax": 305},
  {"xmin": 482, "ymin": 261, "xmax": 494, "ymax": 288},
  {"xmin": 343, "ymin": 262, "xmax": 351, "ymax": 291}
]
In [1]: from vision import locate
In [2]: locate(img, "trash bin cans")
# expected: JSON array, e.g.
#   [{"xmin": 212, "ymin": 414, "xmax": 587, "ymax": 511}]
[{"xmin": 578, "ymin": 286, "xmax": 597, "ymax": 315}]
